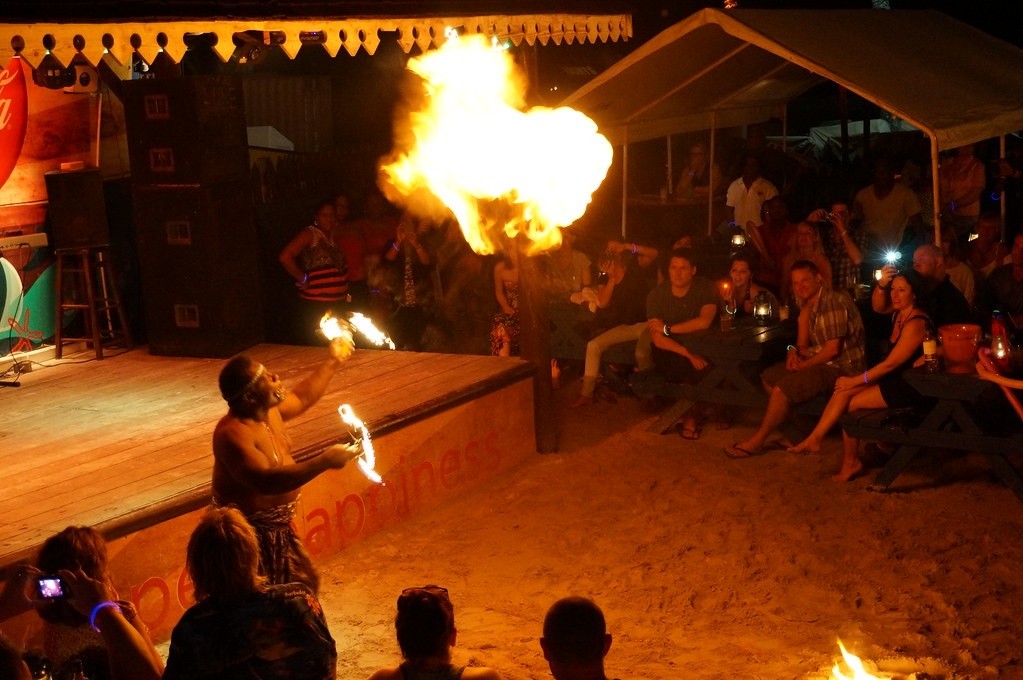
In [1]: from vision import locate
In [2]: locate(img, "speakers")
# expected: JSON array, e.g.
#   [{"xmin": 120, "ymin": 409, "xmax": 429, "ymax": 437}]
[
  {"xmin": 122, "ymin": 74, "xmax": 267, "ymax": 358},
  {"xmin": 44, "ymin": 165, "xmax": 111, "ymax": 251}
]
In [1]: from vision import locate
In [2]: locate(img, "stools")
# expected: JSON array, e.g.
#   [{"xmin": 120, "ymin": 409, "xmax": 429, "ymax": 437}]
[{"xmin": 52, "ymin": 246, "xmax": 133, "ymax": 361}]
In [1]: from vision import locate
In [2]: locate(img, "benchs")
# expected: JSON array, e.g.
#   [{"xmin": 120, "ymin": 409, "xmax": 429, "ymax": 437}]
[
  {"xmin": 623, "ymin": 365, "xmax": 829, "ymax": 410},
  {"xmin": 837, "ymin": 400, "xmax": 913, "ymax": 441}
]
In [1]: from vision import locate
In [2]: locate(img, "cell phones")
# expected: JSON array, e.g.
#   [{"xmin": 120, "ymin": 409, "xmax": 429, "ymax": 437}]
[{"xmin": 403, "ymin": 219, "xmax": 414, "ymax": 234}]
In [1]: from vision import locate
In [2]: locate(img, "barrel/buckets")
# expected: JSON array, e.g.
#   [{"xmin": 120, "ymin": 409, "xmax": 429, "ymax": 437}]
[{"xmin": 937, "ymin": 324, "xmax": 982, "ymax": 373}]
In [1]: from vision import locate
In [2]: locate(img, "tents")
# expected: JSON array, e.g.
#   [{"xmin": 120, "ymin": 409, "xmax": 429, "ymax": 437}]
[{"xmin": 554, "ymin": 6, "xmax": 1023, "ymax": 248}]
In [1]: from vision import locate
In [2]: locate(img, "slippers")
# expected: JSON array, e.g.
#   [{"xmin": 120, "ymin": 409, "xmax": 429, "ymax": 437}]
[
  {"xmin": 720, "ymin": 441, "xmax": 761, "ymax": 460},
  {"xmin": 675, "ymin": 421, "xmax": 698, "ymax": 440}
]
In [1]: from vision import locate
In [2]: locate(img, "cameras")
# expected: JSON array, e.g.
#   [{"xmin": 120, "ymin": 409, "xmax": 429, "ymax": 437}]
[
  {"xmin": 36, "ymin": 577, "xmax": 71, "ymax": 600},
  {"xmin": 825, "ymin": 212, "xmax": 835, "ymax": 222},
  {"xmin": 606, "ymin": 249, "xmax": 616, "ymax": 263}
]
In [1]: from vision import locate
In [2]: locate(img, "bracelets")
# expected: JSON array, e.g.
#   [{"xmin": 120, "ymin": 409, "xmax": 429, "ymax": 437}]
[
  {"xmin": 90, "ymin": 602, "xmax": 122, "ymax": 633},
  {"xmin": 300, "ymin": 274, "xmax": 307, "ymax": 284},
  {"xmin": 878, "ymin": 282, "xmax": 886, "ymax": 290},
  {"xmin": 864, "ymin": 371, "xmax": 868, "ymax": 383},
  {"xmin": 688, "ymin": 173, "xmax": 696, "ymax": 176},
  {"xmin": 728, "ymin": 222, "xmax": 735, "ymax": 227},
  {"xmin": 726, "ymin": 305, "xmax": 737, "ymax": 314},
  {"xmin": 664, "ymin": 325, "xmax": 669, "ymax": 336},
  {"xmin": 631, "ymin": 244, "xmax": 636, "ymax": 253},
  {"xmin": 415, "ymin": 244, "xmax": 422, "ymax": 249},
  {"xmin": 952, "ymin": 201, "xmax": 958, "ymax": 211},
  {"xmin": 372, "ymin": 290, "xmax": 380, "ymax": 293},
  {"xmin": 667, "ymin": 324, "xmax": 672, "ymax": 335},
  {"xmin": 841, "ymin": 230, "xmax": 847, "ymax": 236},
  {"xmin": 991, "ymin": 192, "xmax": 1000, "ymax": 200},
  {"xmin": 787, "ymin": 345, "xmax": 797, "ymax": 350},
  {"xmin": 393, "ymin": 243, "xmax": 401, "ymax": 252}
]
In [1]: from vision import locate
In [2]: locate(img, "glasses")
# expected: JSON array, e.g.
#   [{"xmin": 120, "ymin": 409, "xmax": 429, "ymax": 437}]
[
  {"xmin": 691, "ymin": 150, "xmax": 710, "ymax": 159},
  {"xmin": 402, "ymin": 587, "xmax": 448, "ymax": 596}
]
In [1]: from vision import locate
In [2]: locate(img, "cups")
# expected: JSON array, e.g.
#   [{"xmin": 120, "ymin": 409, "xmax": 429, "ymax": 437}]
[
  {"xmin": 779, "ymin": 305, "xmax": 789, "ymax": 322},
  {"xmin": 849, "ymin": 284, "xmax": 865, "ymax": 300}
]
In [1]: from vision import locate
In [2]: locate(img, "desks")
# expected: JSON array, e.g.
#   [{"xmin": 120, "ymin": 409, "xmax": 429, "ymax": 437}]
[
  {"xmin": 548, "ymin": 301, "xmax": 628, "ymax": 393},
  {"xmin": 649, "ymin": 307, "xmax": 807, "ymax": 457},
  {"xmin": 868, "ymin": 349, "xmax": 1023, "ymax": 502}
]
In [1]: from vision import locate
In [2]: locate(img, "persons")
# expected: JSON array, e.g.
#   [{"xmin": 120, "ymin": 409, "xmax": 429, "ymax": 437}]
[
  {"xmin": 0, "ymin": 525, "xmax": 166, "ymax": 680},
  {"xmin": 211, "ymin": 338, "xmax": 355, "ymax": 597},
  {"xmin": 367, "ymin": 584, "xmax": 502, "ymax": 680},
  {"xmin": 540, "ymin": 596, "xmax": 621, "ymax": 680},
  {"xmin": 278, "ymin": 125, "xmax": 1023, "ymax": 483},
  {"xmin": 162, "ymin": 507, "xmax": 337, "ymax": 680}
]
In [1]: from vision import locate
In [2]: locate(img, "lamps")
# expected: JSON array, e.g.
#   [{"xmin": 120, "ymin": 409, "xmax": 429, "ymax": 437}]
[{"xmin": 750, "ymin": 298, "xmax": 776, "ymax": 328}]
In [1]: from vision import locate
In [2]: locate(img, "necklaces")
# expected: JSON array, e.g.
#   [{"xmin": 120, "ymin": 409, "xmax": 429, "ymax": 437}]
[
  {"xmin": 978, "ymin": 247, "xmax": 990, "ymax": 263},
  {"xmin": 898, "ymin": 309, "xmax": 914, "ymax": 331}
]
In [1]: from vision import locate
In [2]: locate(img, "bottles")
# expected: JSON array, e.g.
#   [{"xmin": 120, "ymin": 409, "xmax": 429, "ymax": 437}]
[{"xmin": 922, "ymin": 330, "xmax": 939, "ymax": 373}]
